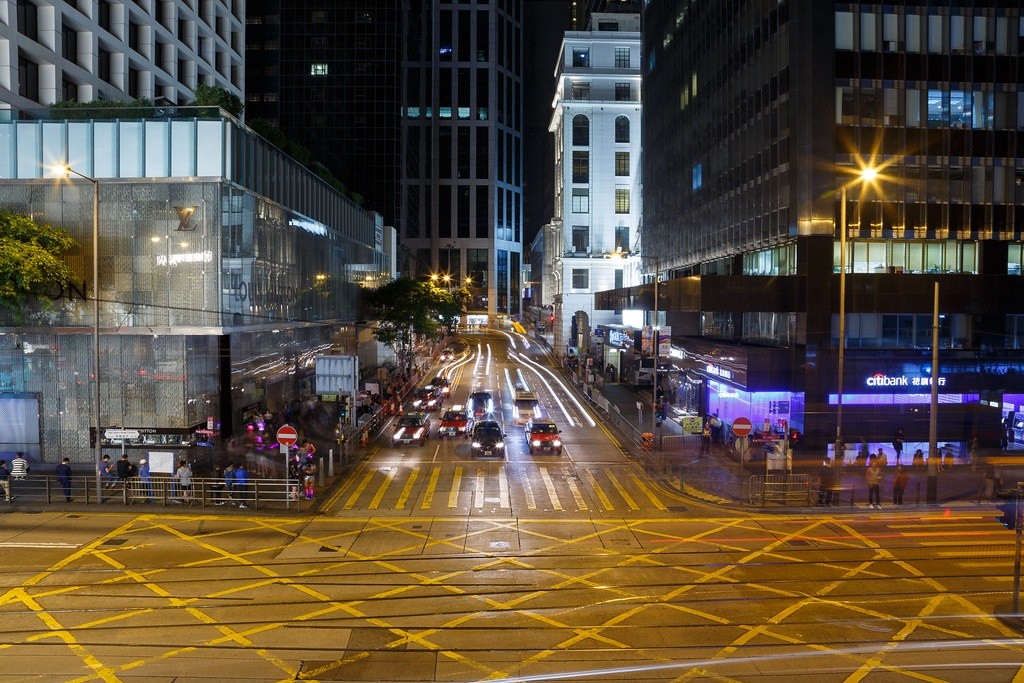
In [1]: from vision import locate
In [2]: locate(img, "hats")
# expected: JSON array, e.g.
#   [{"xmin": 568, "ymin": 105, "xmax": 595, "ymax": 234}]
[
  {"xmin": 712, "ymin": 412, "xmax": 718, "ymax": 417},
  {"xmin": 139, "ymin": 460, "xmax": 147, "ymax": 465}
]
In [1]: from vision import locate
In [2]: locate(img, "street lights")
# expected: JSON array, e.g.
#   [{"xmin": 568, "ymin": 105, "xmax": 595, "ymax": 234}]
[
  {"xmin": 831, "ymin": 170, "xmax": 877, "ymax": 506},
  {"xmin": 53, "ymin": 163, "xmax": 102, "ymax": 505},
  {"xmin": 608, "ymin": 253, "xmax": 659, "ymax": 433}
]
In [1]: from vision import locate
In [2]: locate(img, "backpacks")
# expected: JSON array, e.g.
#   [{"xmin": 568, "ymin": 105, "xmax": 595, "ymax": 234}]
[{"xmin": 224, "ymin": 469, "xmax": 235, "ymax": 487}]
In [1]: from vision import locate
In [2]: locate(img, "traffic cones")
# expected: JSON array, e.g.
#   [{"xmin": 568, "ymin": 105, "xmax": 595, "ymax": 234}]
[
  {"xmin": 398, "ymin": 402, "xmax": 404, "ymax": 416},
  {"xmin": 389, "ymin": 400, "xmax": 397, "ymax": 415},
  {"xmin": 360, "ymin": 431, "xmax": 366, "ymax": 446},
  {"xmin": 365, "ymin": 427, "xmax": 370, "ymax": 444}
]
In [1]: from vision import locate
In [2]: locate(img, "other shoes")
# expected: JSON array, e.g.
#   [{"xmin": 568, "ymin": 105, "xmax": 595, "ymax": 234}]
[
  {"xmin": 243, "ymin": 502, "xmax": 248, "ymax": 506},
  {"xmin": 187, "ymin": 502, "xmax": 192, "ymax": 504},
  {"xmin": 111, "ymin": 484, "xmax": 118, "ymax": 489},
  {"xmin": 869, "ymin": 504, "xmax": 874, "ymax": 509},
  {"xmin": 215, "ymin": 503, "xmax": 222, "ymax": 505},
  {"xmin": 147, "ymin": 500, "xmax": 151, "ymax": 503},
  {"xmin": 122, "ymin": 486, "xmax": 125, "ymax": 490},
  {"xmin": 181, "ymin": 499, "xmax": 185, "ymax": 504},
  {"xmin": 10, "ymin": 496, "xmax": 16, "ymax": 500},
  {"xmin": 232, "ymin": 503, "xmax": 236, "ymax": 505},
  {"xmin": 5, "ymin": 497, "xmax": 10, "ymax": 501},
  {"xmin": 877, "ymin": 504, "xmax": 881, "ymax": 509},
  {"xmin": 105, "ymin": 484, "xmax": 109, "ymax": 489},
  {"xmin": 66, "ymin": 498, "xmax": 73, "ymax": 502},
  {"xmin": 222, "ymin": 502, "xmax": 225, "ymax": 504},
  {"xmin": 144, "ymin": 498, "xmax": 149, "ymax": 503}
]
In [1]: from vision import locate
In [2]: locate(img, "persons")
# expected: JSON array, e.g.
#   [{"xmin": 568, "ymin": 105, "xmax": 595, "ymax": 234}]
[
  {"xmin": 851, "ymin": 441, "xmax": 886, "ymax": 506},
  {"xmin": 0, "ymin": 460, "xmax": 17, "ymax": 502},
  {"xmin": 10, "ymin": 451, "xmax": 31, "ymax": 481},
  {"xmin": 892, "ymin": 428, "xmax": 904, "ymax": 466},
  {"xmin": 561, "ymin": 351, "xmax": 616, "ymax": 383},
  {"xmin": 175, "ymin": 460, "xmax": 194, "ymax": 504},
  {"xmin": 98, "ymin": 454, "xmax": 119, "ymax": 489},
  {"xmin": 137, "ymin": 459, "xmax": 154, "ymax": 503},
  {"xmin": 943, "ymin": 448, "xmax": 955, "ymax": 471},
  {"xmin": 911, "ymin": 449, "xmax": 927, "ymax": 472},
  {"xmin": 891, "ymin": 464, "xmax": 909, "ymax": 506},
  {"xmin": 56, "ymin": 457, "xmax": 73, "ymax": 502},
  {"xmin": 210, "ymin": 464, "xmax": 226, "ymax": 505},
  {"xmin": 223, "ymin": 461, "xmax": 238, "ymax": 506},
  {"xmin": 233, "ymin": 464, "xmax": 250, "ymax": 508},
  {"xmin": 833, "ymin": 442, "xmax": 848, "ymax": 465},
  {"xmin": 288, "ymin": 460, "xmax": 301, "ymax": 501},
  {"xmin": 816, "ymin": 457, "xmax": 843, "ymax": 506},
  {"xmin": 116, "ymin": 454, "xmax": 136, "ymax": 490},
  {"xmin": 343, "ymin": 361, "xmax": 421, "ymax": 435},
  {"xmin": 968, "ymin": 431, "xmax": 981, "ymax": 472},
  {"xmin": 300, "ymin": 440, "xmax": 316, "ymax": 462},
  {"xmin": 998, "ymin": 417, "xmax": 1011, "ymax": 451},
  {"xmin": 696, "ymin": 420, "xmax": 713, "ymax": 458},
  {"xmin": 710, "ymin": 414, "xmax": 721, "ymax": 444},
  {"xmin": 981, "ymin": 457, "xmax": 1002, "ymax": 498},
  {"xmin": 932, "ymin": 448, "xmax": 945, "ymax": 472}
]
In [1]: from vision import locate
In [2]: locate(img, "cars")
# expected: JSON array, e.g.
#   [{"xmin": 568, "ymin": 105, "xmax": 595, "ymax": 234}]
[
  {"xmin": 525, "ymin": 418, "xmax": 563, "ymax": 456},
  {"xmin": 428, "ymin": 377, "xmax": 451, "ymax": 398},
  {"xmin": 469, "ymin": 392, "xmax": 493, "ymax": 418},
  {"xmin": 414, "ymin": 385, "xmax": 443, "ymax": 411},
  {"xmin": 438, "ymin": 409, "xmax": 475, "ymax": 440},
  {"xmin": 392, "ymin": 412, "xmax": 431, "ymax": 447},
  {"xmin": 469, "ymin": 420, "xmax": 508, "ymax": 459}
]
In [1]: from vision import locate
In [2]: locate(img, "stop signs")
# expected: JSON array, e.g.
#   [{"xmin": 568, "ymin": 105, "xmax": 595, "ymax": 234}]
[
  {"xmin": 275, "ymin": 426, "xmax": 298, "ymax": 447},
  {"xmin": 731, "ymin": 417, "xmax": 753, "ymax": 437}
]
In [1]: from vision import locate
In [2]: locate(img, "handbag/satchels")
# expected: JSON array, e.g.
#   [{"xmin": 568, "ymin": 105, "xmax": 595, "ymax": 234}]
[{"xmin": 892, "ymin": 480, "xmax": 897, "ymax": 488}]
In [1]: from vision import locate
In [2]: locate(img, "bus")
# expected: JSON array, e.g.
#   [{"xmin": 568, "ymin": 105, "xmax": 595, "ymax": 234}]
[{"xmin": 512, "ymin": 388, "xmax": 540, "ymax": 428}]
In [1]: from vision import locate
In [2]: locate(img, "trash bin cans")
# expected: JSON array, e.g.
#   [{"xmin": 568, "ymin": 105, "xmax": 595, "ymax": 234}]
[
  {"xmin": 381, "ymin": 399, "xmax": 388, "ymax": 414},
  {"xmin": 642, "ymin": 433, "xmax": 653, "ymax": 451}
]
